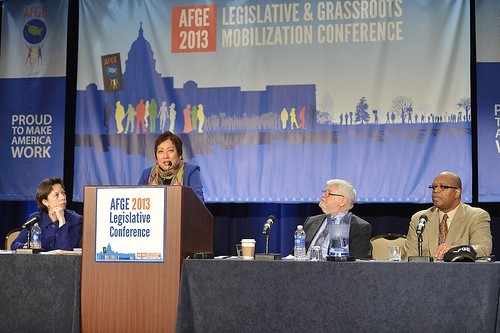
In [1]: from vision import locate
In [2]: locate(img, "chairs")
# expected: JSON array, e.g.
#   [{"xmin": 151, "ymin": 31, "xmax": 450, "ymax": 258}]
[
  {"xmin": 370, "ymin": 234, "xmax": 409, "ymax": 261},
  {"xmin": 5, "ymin": 228, "xmax": 25, "ymax": 251}
]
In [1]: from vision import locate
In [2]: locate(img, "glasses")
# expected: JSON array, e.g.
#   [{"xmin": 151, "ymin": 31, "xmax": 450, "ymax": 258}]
[
  {"xmin": 322, "ymin": 189, "xmax": 345, "ymax": 198},
  {"xmin": 428, "ymin": 184, "xmax": 458, "ymax": 191}
]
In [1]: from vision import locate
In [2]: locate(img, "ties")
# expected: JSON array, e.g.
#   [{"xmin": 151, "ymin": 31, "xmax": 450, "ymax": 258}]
[
  {"xmin": 310, "ymin": 214, "xmax": 337, "ymax": 260},
  {"xmin": 438, "ymin": 213, "xmax": 449, "ymax": 245}
]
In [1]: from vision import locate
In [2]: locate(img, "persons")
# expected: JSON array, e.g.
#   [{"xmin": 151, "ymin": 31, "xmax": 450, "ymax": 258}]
[
  {"xmin": 401, "ymin": 172, "xmax": 493, "ymax": 259},
  {"xmin": 291, "ymin": 179, "xmax": 372, "ymax": 259},
  {"xmin": 138, "ymin": 132, "xmax": 205, "ymax": 203},
  {"xmin": 11, "ymin": 178, "xmax": 83, "ymax": 252}
]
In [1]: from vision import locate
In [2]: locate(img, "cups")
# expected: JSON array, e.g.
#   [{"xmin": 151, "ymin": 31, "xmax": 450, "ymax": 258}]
[
  {"xmin": 389, "ymin": 246, "xmax": 401, "ymax": 261},
  {"xmin": 237, "ymin": 244, "xmax": 243, "ymax": 260},
  {"xmin": 241, "ymin": 239, "xmax": 256, "ymax": 259},
  {"xmin": 309, "ymin": 246, "xmax": 322, "ymax": 261}
]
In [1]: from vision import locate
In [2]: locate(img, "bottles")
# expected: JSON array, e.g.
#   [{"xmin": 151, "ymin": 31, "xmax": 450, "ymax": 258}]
[
  {"xmin": 294, "ymin": 225, "xmax": 306, "ymax": 260},
  {"xmin": 31, "ymin": 222, "xmax": 41, "ymax": 249}
]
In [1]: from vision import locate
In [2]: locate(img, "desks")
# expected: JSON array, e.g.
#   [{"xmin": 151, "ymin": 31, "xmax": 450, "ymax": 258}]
[
  {"xmin": 176, "ymin": 258, "xmax": 500, "ymax": 333},
  {"xmin": 0, "ymin": 251, "xmax": 83, "ymax": 333}
]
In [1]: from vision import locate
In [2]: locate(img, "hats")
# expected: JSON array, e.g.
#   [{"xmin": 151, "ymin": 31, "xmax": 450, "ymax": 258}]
[{"xmin": 444, "ymin": 245, "xmax": 477, "ymax": 263}]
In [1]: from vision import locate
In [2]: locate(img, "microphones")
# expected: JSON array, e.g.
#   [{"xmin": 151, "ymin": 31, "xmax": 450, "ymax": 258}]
[
  {"xmin": 162, "ymin": 163, "xmax": 172, "ymax": 185},
  {"xmin": 262, "ymin": 215, "xmax": 275, "ymax": 234},
  {"xmin": 22, "ymin": 215, "xmax": 41, "ymax": 229},
  {"xmin": 416, "ymin": 214, "xmax": 428, "ymax": 235}
]
